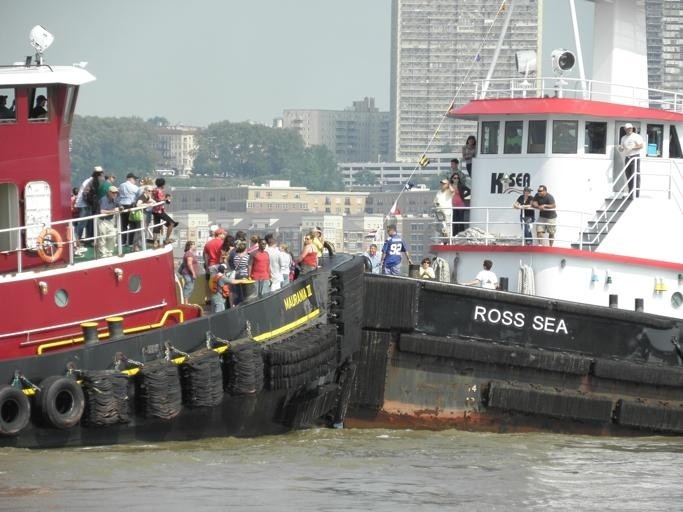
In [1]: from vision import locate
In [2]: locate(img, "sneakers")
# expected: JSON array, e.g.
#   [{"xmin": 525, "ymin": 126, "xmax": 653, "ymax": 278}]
[
  {"xmin": 77, "ymin": 247, "xmax": 88, "ymax": 252},
  {"xmin": 99, "ymin": 238, "xmax": 173, "ymax": 258}
]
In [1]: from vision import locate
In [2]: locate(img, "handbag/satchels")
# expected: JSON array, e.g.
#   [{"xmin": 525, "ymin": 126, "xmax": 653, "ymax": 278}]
[
  {"xmin": 303, "ymin": 252, "xmax": 317, "ymax": 266},
  {"xmin": 128, "ymin": 209, "xmax": 143, "ymax": 222},
  {"xmin": 178, "ymin": 263, "xmax": 183, "ymax": 275}
]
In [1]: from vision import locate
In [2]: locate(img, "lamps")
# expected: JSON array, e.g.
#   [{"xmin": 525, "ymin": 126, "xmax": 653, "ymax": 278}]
[
  {"xmin": 25, "ymin": 22, "xmax": 54, "ymax": 67},
  {"xmin": 549, "ymin": 48, "xmax": 578, "ymax": 99},
  {"xmin": 512, "ymin": 48, "xmax": 538, "ymax": 100}
]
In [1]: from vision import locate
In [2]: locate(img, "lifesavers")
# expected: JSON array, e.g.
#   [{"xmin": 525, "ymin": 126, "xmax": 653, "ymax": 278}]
[{"xmin": 37, "ymin": 229, "xmax": 63, "ymax": 263}]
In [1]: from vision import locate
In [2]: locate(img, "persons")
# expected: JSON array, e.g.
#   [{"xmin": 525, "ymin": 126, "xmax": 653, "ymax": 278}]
[
  {"xmin": 505, "ymin": 127, "xmax": 522, "ymax": 153},
  {"xmin": 0, "ymin": 95, "xmax": 15, "ymax": 119},
  {"xmin": 556, "ymin": 124, "xmax": 577, "ymax": 154},
  {"xmin": 449, "ymin": 173, "xmax": 471, "ymax": 237},
  {"xmin": 462, "ymin": 135, "xmax": 477, "ymax": 179},
  {"xmin": 70, "ymin": 166, "xmax": 179, "ymax": 253},
  {"xmin": 513, "ymin": 187, "xmax": 536, "ymax": 246},
  {"xmin": 618, "ymin": 122, "xmax": 643, "ymax": 200},
  {"xmin": 450, "ymin": 159, "xmax": 466, "ymax": 184},
  {"xmin": 459, "ymin": 260, "xmax": 499, "ymax": 290},
  {"xmin": 532, "ymin": 185, "xmax": 557, "ymax": 246},
  {"xmin": 432, "ymin": 179, "xmax": 455, "ymax": 245},
  {"xmin": 30, "ymin": 95, "xmax": 48, "ymax": 118},
  {"xmin": 378, "ymin": 224, "xmax": 413, "ymax": 276},
  {"xmin": 362, "ymin": 244, "xmax": 380, "ymax": 275},
  {"xmin": 418, "ymin": 258, "xmax": 436, "ymax": 280},
  {"xmin": 180, "ymin": 224, "xmax": 334, "ymax": 312}
]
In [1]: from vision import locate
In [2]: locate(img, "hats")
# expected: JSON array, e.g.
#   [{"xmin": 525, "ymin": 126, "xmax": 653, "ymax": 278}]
[
  {"xmin": 126, "ymin": 173, "xmax": 137, "ymax": 179},
  {"xmin": 624, "ymin": 123, "xmax": 632, "ymax": 129},
  {"xmin": 524, "ymin": 187, "xmax": 532, "ymax": 192},
  {"xmin": 215, "ymin": 229, "xmax": 227, "ymax": 234},
  {"xmin": 217, "ymin": 263, "xmax": 227, "ymax": 271},
  {"xmin": 109, "ymin": 186, "xmax": 118, "ymax": 193},
  {"xmin": 439, "ymin": 179, "xmax": 448, "ymax": 183}
]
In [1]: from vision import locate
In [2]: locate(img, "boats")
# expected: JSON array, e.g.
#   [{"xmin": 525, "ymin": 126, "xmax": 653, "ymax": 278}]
[
  {"xmin": 0, "ymin": 27, "xmax": 370, "ymax": 444},
  {"xmin": 338, "ymin": 1, "xmax": 683, "ymax": 436}
]
